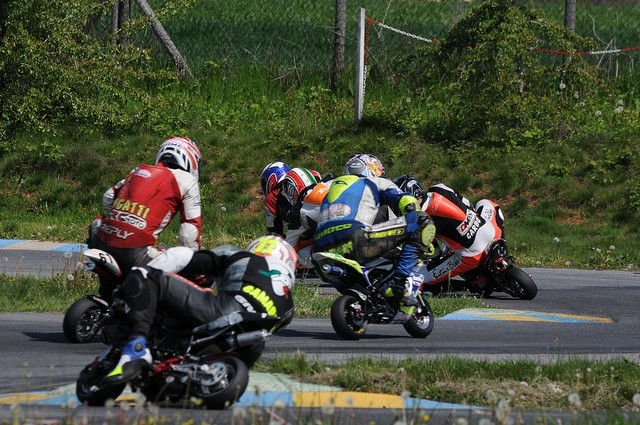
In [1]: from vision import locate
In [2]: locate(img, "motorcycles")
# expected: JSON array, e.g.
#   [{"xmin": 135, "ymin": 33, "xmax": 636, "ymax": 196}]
[
  {"xmin": 460, "ymin": 237, "xmax": 539, "ymax": 299},
  {"xmin": 310, "ymin": 237, "xmax": 435, "ymax": 340},
  {"xmin": 62, "ymin": 249, "xmax": 130, "ymax": 343},
  {"xmin": 76, "ymin": 308, "xmax": 281, "ymax": 411}
]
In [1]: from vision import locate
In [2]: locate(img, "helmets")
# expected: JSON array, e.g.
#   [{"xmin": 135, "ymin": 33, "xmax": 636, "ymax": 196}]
[
  {"xmin": 261, "ymin": 162, "xmax": 290, "ymax": 197},
  {"xmin": 282, "ymin": 167, "xmax": 321, "ymax": 207},
  {"xmin": 245, "ymin": 235, "xmax": 298, "ymax": 274},
  {"xmin": 156, "ymin": 136, "xmax": 201, "ymax": 183},
  {"xmin": 391, "ymin": 174, "xmax": 424, "ymax": 199},
  {"xmin": 344, "ymin": 153, "xmax": 385, "ymax": 179}
]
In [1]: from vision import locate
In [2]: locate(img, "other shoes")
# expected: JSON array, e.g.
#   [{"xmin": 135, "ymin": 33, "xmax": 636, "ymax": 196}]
[{"xmin": 465, "ymin": 276, "xmax": 489, "ymax": 295}]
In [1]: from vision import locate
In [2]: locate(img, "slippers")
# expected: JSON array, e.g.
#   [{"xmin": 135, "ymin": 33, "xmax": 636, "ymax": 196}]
[{"xmin": 102, "ymin": 334, "xmax": 152, "ymax": 386}]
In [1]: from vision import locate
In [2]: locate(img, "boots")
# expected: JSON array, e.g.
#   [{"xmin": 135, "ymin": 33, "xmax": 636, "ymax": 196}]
[{"xmin": 379, "ymin": 275, "xmax": 408, "ymax": 299}]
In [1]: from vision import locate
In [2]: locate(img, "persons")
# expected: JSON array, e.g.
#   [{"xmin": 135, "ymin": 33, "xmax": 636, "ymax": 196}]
[
  {"xmin": 101, "ymin": 236, "xmax": 297, "ymax": 383},
  {"xmin": 260, "ymin": 152, "xmax": 436, "ymax": 307},
  {"xmin": 84, "ymin": 136, "xmax": 215, "ymax": 296},
  {"xmin": 392, "ymin": 175, "xmax": 505, "ymax": 284}
]
